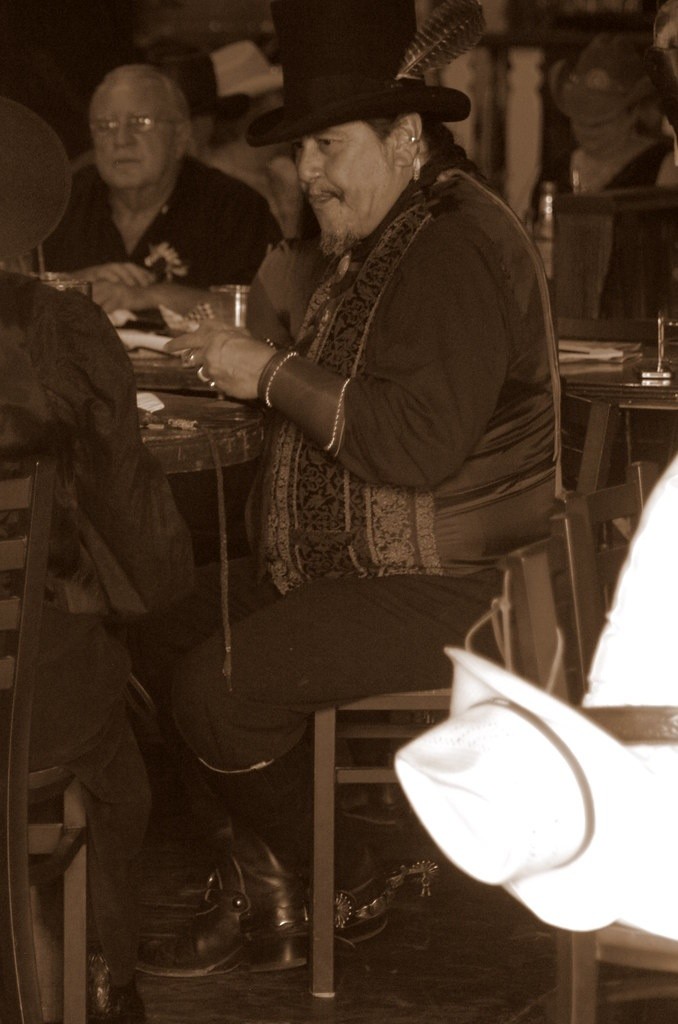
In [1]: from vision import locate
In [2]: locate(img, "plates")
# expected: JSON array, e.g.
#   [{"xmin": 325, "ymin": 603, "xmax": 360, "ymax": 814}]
[{"xmin": 117, "ymin": 333, "xmax": 191, "ymax": 359}]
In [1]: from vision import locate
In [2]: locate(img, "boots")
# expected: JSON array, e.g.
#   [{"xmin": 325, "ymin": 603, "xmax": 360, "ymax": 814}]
[
  {"xmin": 196, "ymin": 747, "xmax": 391, "ymax": 942},
  {"xmin": 133, "ymin": 812, "xmax": 309, "ymax": 977}
]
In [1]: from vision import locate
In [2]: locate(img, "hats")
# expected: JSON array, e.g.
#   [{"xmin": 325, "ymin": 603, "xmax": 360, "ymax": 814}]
[
  {"xmin": 0, "ymin": 98, "xmax": 72, "ymax": 261},
  {"xmin": 392, "ymin": 648, "xmax": 667, "ymax": 932},
  {"xmin": 244, "ymin": 0, "xmax": 471, "ymax": 148},
  {"xmin": 549, "ymin": 32, "xmax": 653, "ymax": 126}
]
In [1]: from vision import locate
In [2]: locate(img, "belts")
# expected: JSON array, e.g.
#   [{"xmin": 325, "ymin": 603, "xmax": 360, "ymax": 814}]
[{"xmin": 582, "ymin": 705, "xmax": 675, "ymax": 744}]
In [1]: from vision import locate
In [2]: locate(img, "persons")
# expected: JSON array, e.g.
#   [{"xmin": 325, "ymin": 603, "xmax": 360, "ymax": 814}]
[
  {"xmin": 532, "ymin": 33, "xmax": 678, "ymax": 231},
  {"xmin": 135, "ymin": 0, "xmax": 561, "ymax": 977},
  {"xmin": 35, "ymin": 61, "xmax": 282, "ymax": 325},
  {"xmin": 0, "ymin": 91, "xmax": 197, "ymax": 1023},
  {"xmin": 142, "ymin": 37, "xmax": 247, "ymax": 153},
  {"xmin": 573, "ymin": 443, "xmax": 678, "ymax": 960}
]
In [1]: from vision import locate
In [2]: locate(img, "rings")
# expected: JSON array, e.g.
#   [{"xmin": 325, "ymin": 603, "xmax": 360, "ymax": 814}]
[{"xmin": 184, "ymin": 348, "xmax": 216, "ymax": 389}]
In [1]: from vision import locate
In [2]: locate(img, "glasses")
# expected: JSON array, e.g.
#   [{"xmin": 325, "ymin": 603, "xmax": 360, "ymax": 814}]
[{"xmin": 87, "ymin": 116, "xmax": 181, "ymax": 135}]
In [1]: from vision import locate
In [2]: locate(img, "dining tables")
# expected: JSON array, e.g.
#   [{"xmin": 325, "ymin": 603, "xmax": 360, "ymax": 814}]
[
  {"xmin": 555, "ymin": 336, "xmax": 678, "ymax": 497},
  {"xmin": 92, "ymin": 308, "xmax": 269, "ymax": 478}
]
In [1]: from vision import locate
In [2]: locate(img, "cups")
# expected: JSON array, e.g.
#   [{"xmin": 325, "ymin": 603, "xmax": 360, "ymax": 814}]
[{"xmin": 210, "ymin": 283, "xmax": 261, "ymax": 338}]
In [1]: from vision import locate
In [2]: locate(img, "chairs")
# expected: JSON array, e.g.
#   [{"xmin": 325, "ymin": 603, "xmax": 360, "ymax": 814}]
[{"xmin": 0, "ymin": 388, "xmax": 678, "ymax": 1024}]
